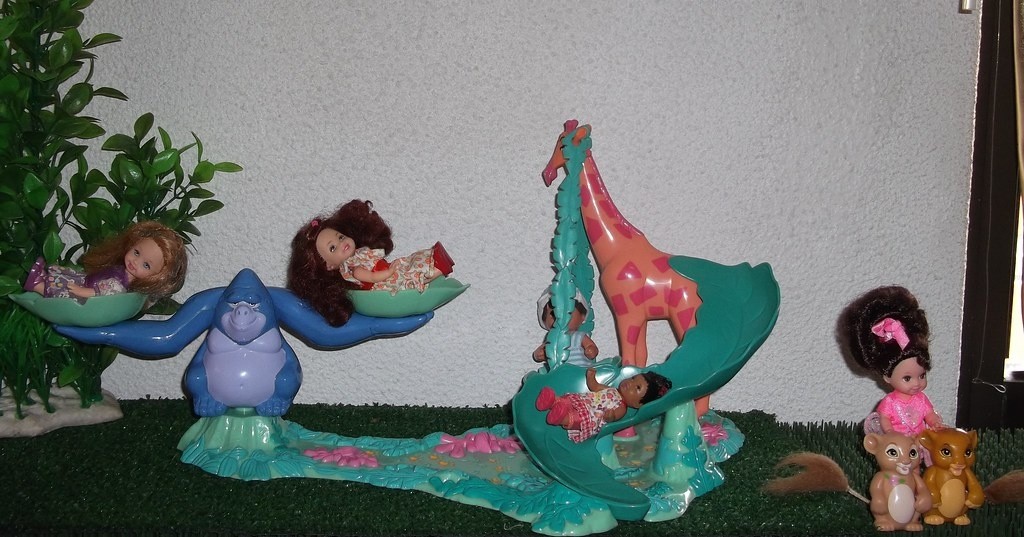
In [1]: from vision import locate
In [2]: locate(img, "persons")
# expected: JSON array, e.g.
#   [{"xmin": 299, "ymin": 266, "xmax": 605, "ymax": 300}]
[
  {"xmin": 285, "ymin": 196, "xmax": 456, "ymax": 329},
  {"xmin": 844, "ymin": 283, "xmax": 950, "ymax": 478},
  {"xmin": 530, "ymin": 365, "xmax": 675, "ymax": 444},
  {"xmin": 531, "ymin": 279, "xmax": 599, "ymax": 370},
  {"xmin": 23, "ymin": 219, "xmax": 191, "ymax": 315}
]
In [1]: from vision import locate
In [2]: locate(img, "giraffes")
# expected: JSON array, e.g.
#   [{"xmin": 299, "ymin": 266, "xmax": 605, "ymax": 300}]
[{"xmin": 541, "ymin": 119, "xmax": 711, "ymax": 421}]
